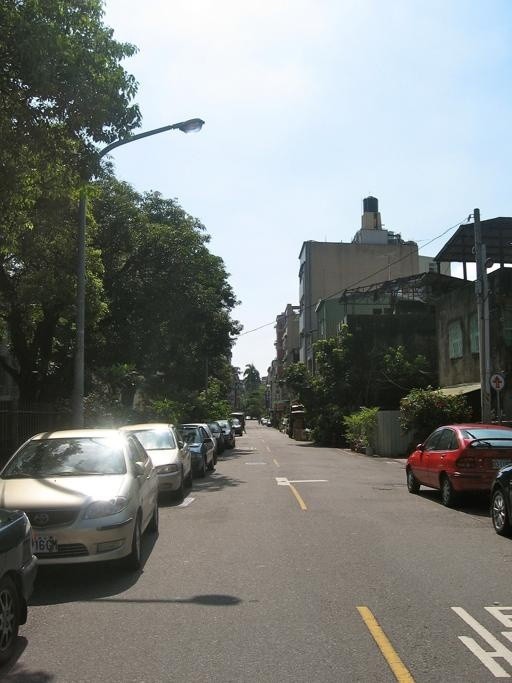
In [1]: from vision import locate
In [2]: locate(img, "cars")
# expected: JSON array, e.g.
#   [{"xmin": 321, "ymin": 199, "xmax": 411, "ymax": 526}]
[{"xmin": 406, "ymin": 426, "xmax": 511, "ymax": 539}]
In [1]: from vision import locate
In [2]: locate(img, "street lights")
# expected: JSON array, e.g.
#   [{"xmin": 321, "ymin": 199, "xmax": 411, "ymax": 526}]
[{"xmin": 71, "ymin": 116, "xmax": 205, "ymax": 430}]
